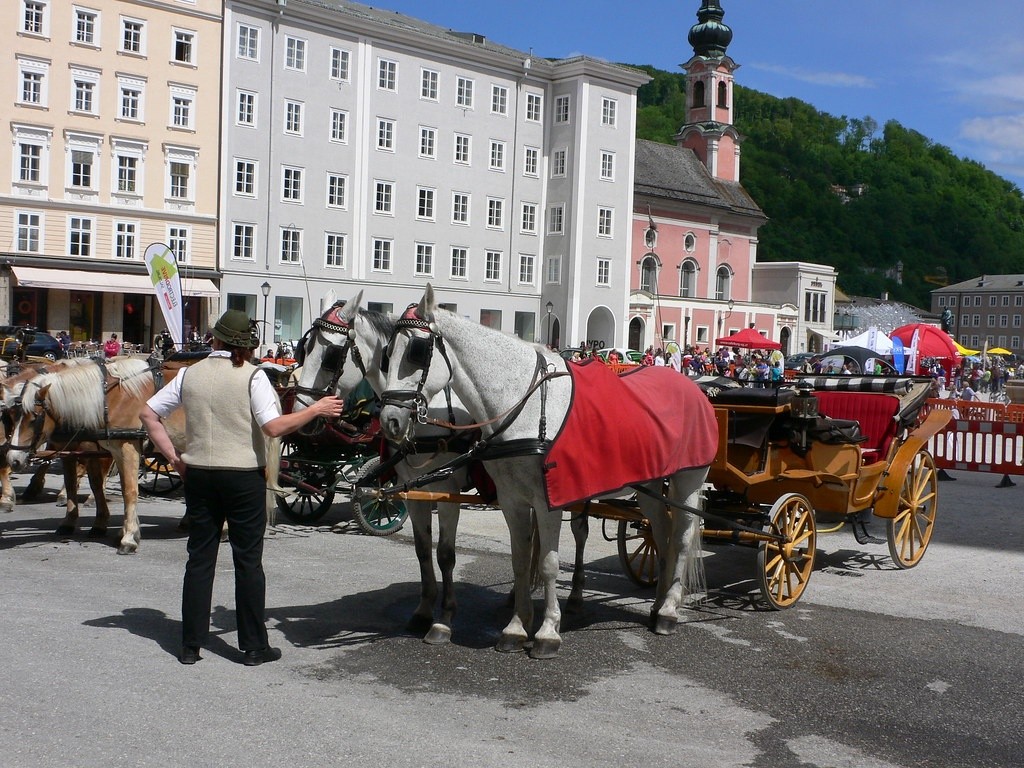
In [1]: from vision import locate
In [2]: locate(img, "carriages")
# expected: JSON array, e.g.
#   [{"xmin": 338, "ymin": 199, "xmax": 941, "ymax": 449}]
[
  {"xmin": 0, "ymin": 338, "xmax": 410, "ymax": 554},
  {"xmin": 291, "ymin": 282, "xmax": 955, "ymax": 659}
]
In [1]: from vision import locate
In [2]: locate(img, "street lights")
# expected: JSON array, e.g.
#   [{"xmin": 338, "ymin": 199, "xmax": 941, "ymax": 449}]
[
  {"xmin": 261, "ymin": 281, "xmax": 272, "ymax": 346},
  {"xmin": 546, "ymin": 300, "xmax": 554, "ymax": 345}
]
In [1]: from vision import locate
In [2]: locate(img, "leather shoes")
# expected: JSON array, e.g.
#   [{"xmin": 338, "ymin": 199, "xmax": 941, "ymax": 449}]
[
  {"xmin": 243, "ymin": 645, "xmax": 282, "ymax": 665},
  {"xmin": 182, "ymin": 643, "xmax": 200, "ymax": 664}
]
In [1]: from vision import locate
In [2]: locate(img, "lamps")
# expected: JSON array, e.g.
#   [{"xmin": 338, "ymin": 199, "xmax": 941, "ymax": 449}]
[{"xmin": 718, "ymin": 299, "xmax": 736, "ymax": 324}]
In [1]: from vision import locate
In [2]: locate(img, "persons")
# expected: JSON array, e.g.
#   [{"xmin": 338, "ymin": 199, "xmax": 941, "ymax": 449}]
[
  {"xmin": 139, "ymin": 307, "xmax": 343, "ymax": 665},
  {"xmin": 874, "ymin": 359, "xmax": 882, "ymax": 375},
  {"xmin": 942, "ymin": 305, "xmax": 952, "ymax": 331},
  {"xmin": 263, "ymin": 349, "xmax": 274, "ymax": 358},
  {"xmin": 203, "ymin": 329, "xmax": 213, "ymax": 347},
  {"xmin": 921, "ymin": 357, "xmax": 935, "ymax": 368},
  {"xmin": 799, "ymin": 358, "xmax": 812, "ymax": 373},
  {"xmin": 893, "ymin": 370, "xmax": 899, "ymax": 375},
  {"xmin": 928, "ymin": 359, "xmax": 1024, "ymax": 401},
  {"xmin": 546, "ymin": 341, "xmax": 784, "ymax": 389},
  {"xmin": 839, "ymin": 364, "xmax": 852, "ymax": 374},
  {"xmin": 187, "ymin": 325, "xmax": 202, "ymax": 342},
  {"xmin": 822, "ymin": 365, "xmax": 834, "ymax": 374},
  {"xmin": 56, "ymin": 331, "xmax": 70, "ymax": 351},
  {"xmin": 991, "ymin": 354, "xmax": 1006, "ymax": 367},
  {"xmin": 104, "ymin": 334, "xmax": 120, "ymax": 359},
  {"xmin": 812, "ymin": 357, "xmax": 821, "ymax": 374}
]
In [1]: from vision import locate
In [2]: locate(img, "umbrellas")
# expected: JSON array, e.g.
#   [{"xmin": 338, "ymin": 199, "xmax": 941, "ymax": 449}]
[
  {"xmin": 986, "ymin": 347, "xmax": 1013, "ymax": 356},
  {"xmin": 952, "ymin": 340, "xmax": 980, "ymax": 356}
]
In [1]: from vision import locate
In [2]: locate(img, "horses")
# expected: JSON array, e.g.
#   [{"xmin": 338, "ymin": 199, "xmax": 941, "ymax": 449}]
[
  {"xmin": 379, "ymin": 282, "xmax": 717, "ymax": 659},
  {"xmin": 292, "ymin": 290, "xmax": 589, "ymax": 644},
  {"xmin": 0, "ymin": 356, "xmax": 281, "ymax": 555}
]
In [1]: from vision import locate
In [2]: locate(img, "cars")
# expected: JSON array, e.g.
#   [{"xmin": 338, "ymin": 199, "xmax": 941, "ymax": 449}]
[
  {"xmin": 559, "ymin": 348, "xmax": 582, "ymax": 362},
  {"xmin": 597, "ymin": 348, "xmax": 643, "ymax": 365},
  {"xmin": 0, "ymin": 331, "xmax": 63, "ymax": 363},
  {"xmin": 783, "ymin": 353, "xmax": 816, "ymax": 370}
]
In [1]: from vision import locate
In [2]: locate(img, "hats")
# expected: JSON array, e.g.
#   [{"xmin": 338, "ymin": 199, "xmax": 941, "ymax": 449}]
[{"xmin": 208, "ymin": 309, "xmax": 261, "ymax": 349}]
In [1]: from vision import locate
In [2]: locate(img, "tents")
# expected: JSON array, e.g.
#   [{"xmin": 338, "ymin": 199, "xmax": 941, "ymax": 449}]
[
  {"xmin": 809, "ymin": 326, "xmax": 919, "ymax": 375},
  {"xmin": 715, "ymin": 329, "xmax": 782, "ymax": 362}
]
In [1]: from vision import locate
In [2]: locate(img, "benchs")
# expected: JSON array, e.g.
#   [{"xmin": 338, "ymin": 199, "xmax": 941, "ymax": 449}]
[
  {"xmin": 701, "ymin": 367, "xmax": 799, "ymax": 469},
  {"xmin": 814, "ymin": 389, "xmax": 908, "ymax": 471}
]
